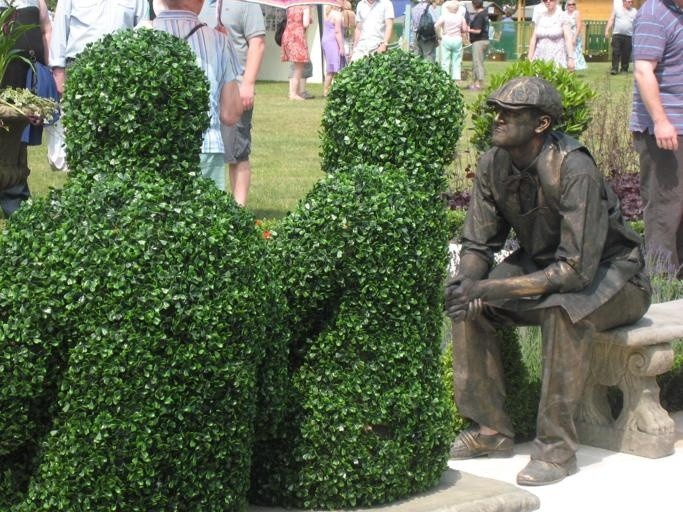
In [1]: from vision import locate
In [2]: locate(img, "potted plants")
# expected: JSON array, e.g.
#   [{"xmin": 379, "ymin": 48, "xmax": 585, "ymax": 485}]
[{"xmin": 0, "ymin": 0, "xmax": 59, "ymax": 191}]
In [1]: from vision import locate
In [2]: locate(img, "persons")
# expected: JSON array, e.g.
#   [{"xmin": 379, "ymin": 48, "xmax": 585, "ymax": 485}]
[
  {"xmin": 439, "ymin": 77, "xmax": 652, "ymax": 488},
  {"xmin": 281, "ymin": 0, "xmax": 515, "ymax": 100},
  {"xmin": 0, "ymin": 0, "xmax": 266, "ymax": 221},
  {"xmin": 605, "ymin": 0, "xmax": 639, "ymax": 76},
  {"xmin": 528, "ymin": 0, "xmax": 585, "ymax": 71},
  {"xmin": 626, "ymin": 0, "xmax": 682, "ymax": 282}
]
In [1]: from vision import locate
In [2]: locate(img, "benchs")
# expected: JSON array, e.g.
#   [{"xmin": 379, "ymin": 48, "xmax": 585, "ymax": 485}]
[{"xmin": 571, "ymin": 297, "xmax": 682, "ymax": 460}]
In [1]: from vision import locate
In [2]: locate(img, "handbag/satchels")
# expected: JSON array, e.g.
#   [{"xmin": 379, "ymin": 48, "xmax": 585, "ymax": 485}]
[
  {"xmin": 288, "ymin": 61, "xmax": 312, "ymax": 78},
  {"xmin": 418, "ymin": 13, "xmax": 436, "ymax": 41},
  {"xmin": 31, "ymin": 62, "xmax": 61, "ymax": 128},
  {"xmin": 275, "ymin": 16, "xmax": 286, "ymax": 46}
]
